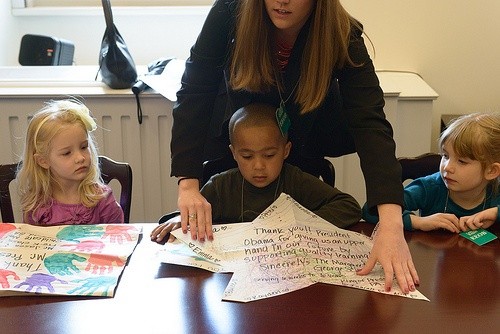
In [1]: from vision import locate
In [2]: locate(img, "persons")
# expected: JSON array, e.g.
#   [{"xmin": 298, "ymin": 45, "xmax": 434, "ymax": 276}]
[
  {"xmin": 15, "ymin": 100, "xmax": 124, "ymax": 228},
  {"xmin": 362, "ymin": 113, "xmax": 500, "ymax": 233},
  {"xmin": 151, "ymin": 105, "xmax": 362, "ymax": 242},
  {"xmin": 171, "ymin": 0, "xmax": 420, "ymax": 294}
]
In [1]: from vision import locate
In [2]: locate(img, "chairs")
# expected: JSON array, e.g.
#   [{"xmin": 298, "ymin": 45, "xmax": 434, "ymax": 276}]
[
  {"xmin": 201, "ymin": 155, "xmax": 336, "ymax": 190},
  {"xmin": 396, "ymin": 152, "xmax": 443, "ymax": 184},
  {"xmin": 0, "ymin": 155, "xmax": 133, "ymax": 224}
]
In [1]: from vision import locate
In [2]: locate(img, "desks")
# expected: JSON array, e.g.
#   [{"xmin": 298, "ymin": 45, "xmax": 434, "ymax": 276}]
[
  {"xmin": 0, "ymin": 65, "xmax": 440, "ymax": 223},
  {"xmin": 0, "ymin": 220, "xmax": 500, "ymax": 334}
]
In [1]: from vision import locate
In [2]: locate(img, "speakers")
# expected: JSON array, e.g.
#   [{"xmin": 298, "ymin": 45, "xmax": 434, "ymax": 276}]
[{"xmin": 18, "ymin": 34, "xmax": 75, "ymax": 65}]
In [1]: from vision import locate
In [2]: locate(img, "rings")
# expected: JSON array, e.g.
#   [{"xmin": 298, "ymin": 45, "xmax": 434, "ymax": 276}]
[{"xmin": 189, "ymin": 214, "xmax": 196, "ymax": 217}]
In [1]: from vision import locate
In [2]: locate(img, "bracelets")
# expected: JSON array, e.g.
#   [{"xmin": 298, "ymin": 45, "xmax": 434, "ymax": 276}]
[{"xmin": 177, "ymin": 177, "xmax": 201, "ymax": 189}]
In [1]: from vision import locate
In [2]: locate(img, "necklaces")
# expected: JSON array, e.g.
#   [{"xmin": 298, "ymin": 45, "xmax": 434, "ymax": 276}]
[
  {"xmin": 444, "ymin": 188, "xmax": 487, "ymax": 215},
  {"xmin": 241, "ymin": 174, "xmax": 281, "ymax": 222},
  {"xmin": 271, "ymin": 57, "xmax": 301, "ymax": 136}
]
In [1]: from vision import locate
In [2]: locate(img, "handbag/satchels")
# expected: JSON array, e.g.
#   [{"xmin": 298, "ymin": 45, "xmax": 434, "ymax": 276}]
[{"xmin": 94, "ymin": 1, "xmax": 137, "ymax": 90}]
